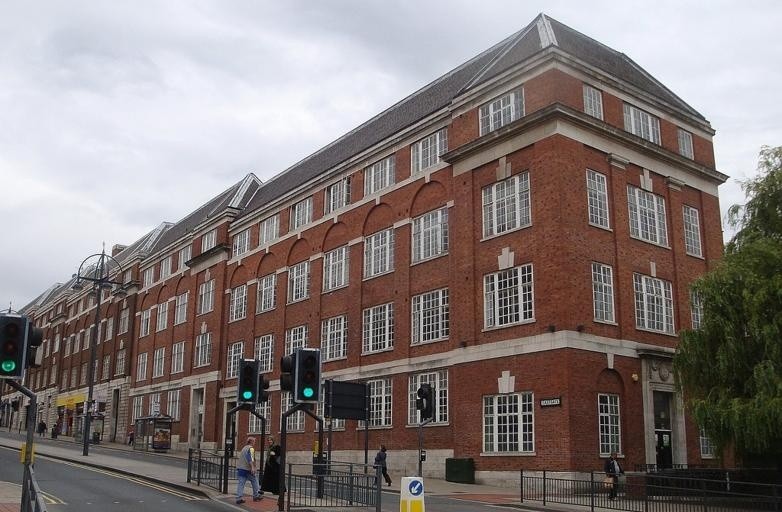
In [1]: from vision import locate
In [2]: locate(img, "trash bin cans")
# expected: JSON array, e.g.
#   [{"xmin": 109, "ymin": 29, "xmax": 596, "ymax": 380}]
[
  {"xmin": 92, "ymin": 432, "xmax": 99, "ymax": 444},
  {"xmin": 446, "ymin": 458, "xmax": 475, "ymax": 483},
  {"xmin": 313, "ymin": 452, "xmax": 327, "ymax": 475}
]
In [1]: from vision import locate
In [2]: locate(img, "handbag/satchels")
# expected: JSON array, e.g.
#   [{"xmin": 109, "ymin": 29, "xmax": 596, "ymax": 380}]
[{"xmin": 604, "ymin": 478, "xmax": 614, "ymax": 488}]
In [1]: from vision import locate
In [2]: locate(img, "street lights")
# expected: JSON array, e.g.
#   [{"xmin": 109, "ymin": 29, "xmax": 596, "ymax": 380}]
[{"xmin": 71, "ymin": 242, "xmax": 126, "ymax": 456}]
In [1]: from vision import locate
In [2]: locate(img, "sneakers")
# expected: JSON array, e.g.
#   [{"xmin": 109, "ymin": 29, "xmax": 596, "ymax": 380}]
[
  {"xmin": 253, "ymin": 495, "xmax": 264, "ymax": 502},
  {"xmin": 236, "ymin": 499, "xmax": 246, "ymax": 505},
  {"xmin": 388, "ymin": 479, "xmax": 392, "ymax": 486}
]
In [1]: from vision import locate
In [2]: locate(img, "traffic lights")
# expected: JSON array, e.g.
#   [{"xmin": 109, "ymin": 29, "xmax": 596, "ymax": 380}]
[
  {"xmin": 280, "ymin": 347, "xmax": 322, "ymax": 404},
  {"xmin": 237, "ymin": 356, "xmax": 269, "ymax": 403},
  {"xmin": 1, "ymin": 312, "xmax": 43, "ymax": 380},
  {"xmin": 417, "ymin": 382, "xmax": 432, "ymax": 419}
]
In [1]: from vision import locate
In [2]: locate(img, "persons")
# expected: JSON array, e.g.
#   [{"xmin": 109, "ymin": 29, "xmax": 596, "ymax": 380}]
[
  {"xmin": 235, "ymin": 437, "xmax": 264, "ymax": 504},
  {"xmin": 128, "ymin": 431, "xmax": 134, "ymax": 446},
  {"xmin": 258, "ymin": 436, "xmax": 287, "ymax": 495},
  {"xmin": 372, "ymin": 445, "xmax": 392, "ymax": 486},
  {"xmin": 604, "ymin": 451, "xmax": 624, "ymax": 501},
  {"xmin": 38, "ymin": 420, "xmax": 46, "ymax": 437}
]
